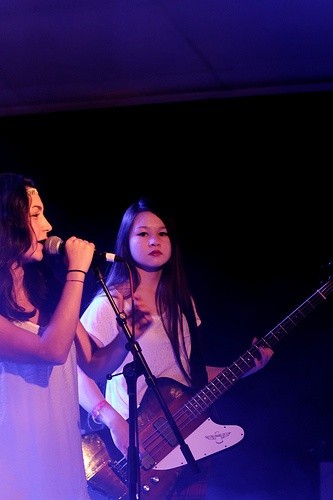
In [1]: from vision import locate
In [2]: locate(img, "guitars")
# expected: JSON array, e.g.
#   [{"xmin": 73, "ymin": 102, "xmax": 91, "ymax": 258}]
[{"xmin": 80, "ymin": 277, "xmax": 329, "ymax": 500}]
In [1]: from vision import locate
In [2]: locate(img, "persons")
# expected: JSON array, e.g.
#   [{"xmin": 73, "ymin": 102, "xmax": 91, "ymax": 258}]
[
  {"xmin": 1, "ymin": 174, "xmax": 153, "ymax": 499},
  {"xmin": 84, "ymin": 196, "xmax": 272, "ymax": 500}
]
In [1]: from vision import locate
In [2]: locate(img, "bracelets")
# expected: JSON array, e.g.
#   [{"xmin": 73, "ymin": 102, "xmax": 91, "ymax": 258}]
[
  {"xmin": 90, "ymin": 398, "xmax": 108, "ymax": 420},
  {"xmin": 65, "ymin": 268, "xmax": 87, "ymax": 276},
  {"xmin": 64, "ymin": 278, "xmax": 85, "ymax": 286}
]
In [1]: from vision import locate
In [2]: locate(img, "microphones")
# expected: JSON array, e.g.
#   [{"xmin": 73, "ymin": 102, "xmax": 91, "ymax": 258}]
[{"xmin": 45, "ymin": 236, "xmax": 122, "ymax": 263}]
